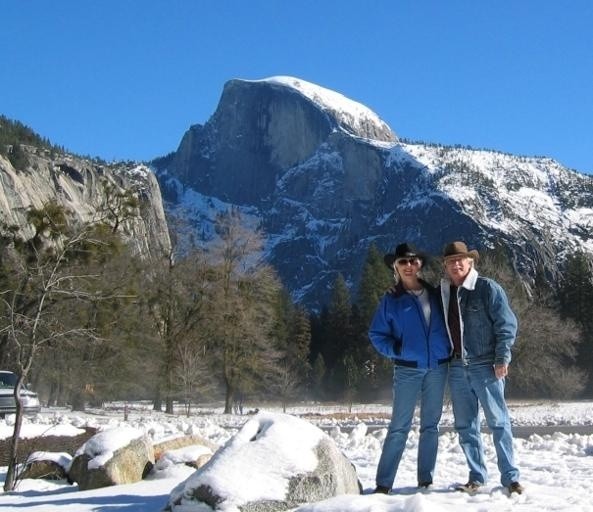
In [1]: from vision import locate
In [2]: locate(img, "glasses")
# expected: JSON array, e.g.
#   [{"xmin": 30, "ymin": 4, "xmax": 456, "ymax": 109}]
[{"xmin": 397, "ymin": 259, "xmax": 418, "ymax": 265}]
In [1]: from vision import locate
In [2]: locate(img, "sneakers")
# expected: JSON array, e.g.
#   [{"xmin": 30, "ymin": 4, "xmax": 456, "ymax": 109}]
[
  {"xmin": 510, "ymin": 482, "xmax": 522, "ymax": 494},
  {"xmin": 455, "ymin": 481, "xmax": 479, "ymax": 492},
  {"xmin": 373, "ymin": 486, "xmax": 392, "ymax": 494},
  {"xmin": 418, "ymin": 481, "xmax": 432, "ymax": 489}
]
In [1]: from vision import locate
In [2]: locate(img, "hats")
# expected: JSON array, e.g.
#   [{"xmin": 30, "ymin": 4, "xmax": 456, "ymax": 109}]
[
  {"xmin": 384, "ymin": 243, "xmax": 427, "ymax": 271},
  {"xmin": 443, "ymin": 242, "xmax": 479, "ymax": 261}
]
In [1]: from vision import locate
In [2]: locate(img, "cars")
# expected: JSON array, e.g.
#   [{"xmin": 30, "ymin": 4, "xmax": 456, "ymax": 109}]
[{"xmin": 0, "ymin": 371, "xmax": 40, "ymax": 417}]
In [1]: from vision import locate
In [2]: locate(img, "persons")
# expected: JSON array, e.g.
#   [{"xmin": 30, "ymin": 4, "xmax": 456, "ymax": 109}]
[
  {"xmin": 368, "ymin": 243, "xmax": 451, "ymax": 494},
  {"xmin": 384, "ymin": 241, "xmax": 525, "ymax": 495}
]
